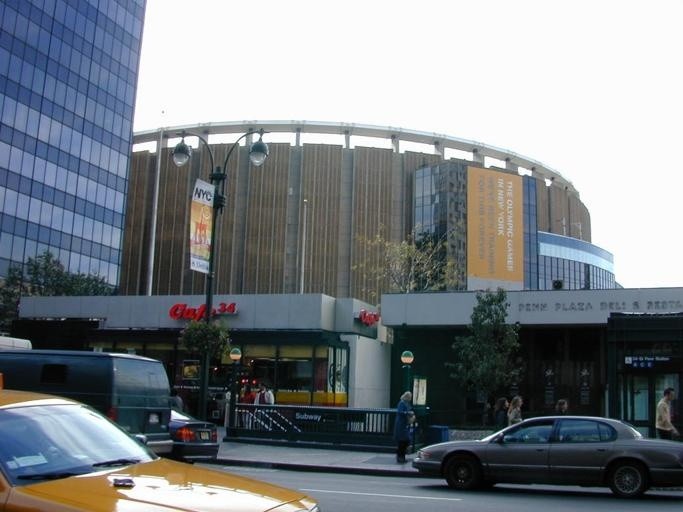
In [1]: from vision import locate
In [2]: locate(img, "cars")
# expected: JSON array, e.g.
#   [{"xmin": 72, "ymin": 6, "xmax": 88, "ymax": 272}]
[
  {"xmin": 169, "ymin": 405, "xmax": 219, "ymax": 464},
  {"xmin": 412, "ymin": 416, "xmax": 683, "ymax": 497},
  {"xmin": 0, "ymin": 390, "xmax": 320, "ymax": 512}
]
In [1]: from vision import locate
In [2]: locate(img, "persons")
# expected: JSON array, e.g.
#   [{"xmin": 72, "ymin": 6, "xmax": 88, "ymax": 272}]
[
  {"xmin": 239, "ymin": 382, "xmax": 275, "ymax": 429},
  {"xmin": 393, "ymin": 391, "xmax": 416, "ymax": 463},
  {"xmin": 480, "ymin": 398, "xmax": 495, "ymax": 427},
  {"xmin": 655, "ymin": 387, "xmax": 680, "ymax": 440},
  {"xmin": 493, "ymin": 397, "xmax": 509, "ymax": 431},
  {"xmin": 507, "ymin": 396, "xmax": 523, "ymax": 426},
  {"xmin": 555, "ymin": 399, "xmax": 568, "ymax": 416}
]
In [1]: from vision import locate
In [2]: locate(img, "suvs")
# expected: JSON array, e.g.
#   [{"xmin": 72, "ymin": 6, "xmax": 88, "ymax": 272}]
[{"xmin": 0, "ymin": 348, "xmax": 173, "ymax": 456}]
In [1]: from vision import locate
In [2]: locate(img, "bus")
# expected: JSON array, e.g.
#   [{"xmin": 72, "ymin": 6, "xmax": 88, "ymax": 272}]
[{"xmin": 172, "ymin": 385, "xmax": 226, "ymax": 417}]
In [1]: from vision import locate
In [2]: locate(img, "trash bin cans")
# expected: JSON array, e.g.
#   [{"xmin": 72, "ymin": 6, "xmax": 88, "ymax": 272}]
[
  {"xmin": 208, "ymin": 400, "xmax": 225, "ymax": 425},
  {"xmin": 425, "ymin": 425, "xmax": 449, "ymax": 445}
]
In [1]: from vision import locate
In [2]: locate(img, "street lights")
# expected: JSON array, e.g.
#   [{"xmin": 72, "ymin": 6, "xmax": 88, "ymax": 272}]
[
  {"xmin": 401, "ymin": 352, "xmax": 416, "ymax": 411},
  {"xmin": 228, "ymin": 348, "xmax": 242, "ymax": 429},
  {"xmin": 172, "ymin": 128, "xmax": 274, "ymax": 418}
]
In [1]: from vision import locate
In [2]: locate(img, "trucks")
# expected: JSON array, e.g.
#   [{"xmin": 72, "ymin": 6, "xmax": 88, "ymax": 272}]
[{"xmin": 0, "ymin": 333, "xmax": 34, "ymax": 353}]
